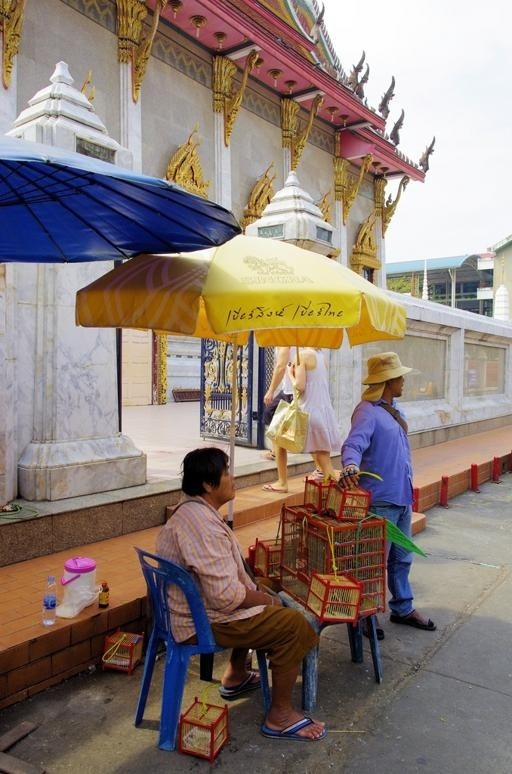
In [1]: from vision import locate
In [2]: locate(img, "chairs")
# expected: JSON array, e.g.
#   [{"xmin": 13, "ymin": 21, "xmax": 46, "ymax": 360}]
[{"xmin": 127, "ymin": 543, "xmax": 284, "ymax": 749}]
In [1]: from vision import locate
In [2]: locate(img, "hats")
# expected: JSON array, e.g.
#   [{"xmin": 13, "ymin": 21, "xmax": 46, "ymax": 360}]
[{"xmin": 362, "ymin": 352, "xmax": 413, "ymax": 384}]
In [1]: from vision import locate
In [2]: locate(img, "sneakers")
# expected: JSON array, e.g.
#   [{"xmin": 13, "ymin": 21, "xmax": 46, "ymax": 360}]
[
  {"xmin": 260, "ymin": 451, "xmax": 276, "ymax": 459},
  {"xmin": 303, "ymin": 471, "xmax": 325, "ymax": 486}
]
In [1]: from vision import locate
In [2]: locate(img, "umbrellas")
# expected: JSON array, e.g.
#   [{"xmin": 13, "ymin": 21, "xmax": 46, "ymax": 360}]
[
  {"xmin": 73, "ymin": 234, "xmax": 407, "ymax": 532},
  {"xmin": 0, "ymin": 129, "xmax": 242, "ymax": 264}
]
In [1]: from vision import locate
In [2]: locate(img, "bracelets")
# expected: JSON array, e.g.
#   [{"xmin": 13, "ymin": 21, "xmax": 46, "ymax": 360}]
[{"xmin": 263, "ymin": 590, "xmax": 276, "ymax": 607}]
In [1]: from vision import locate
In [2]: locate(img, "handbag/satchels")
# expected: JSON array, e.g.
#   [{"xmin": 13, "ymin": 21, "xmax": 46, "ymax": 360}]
[{"xmin": 265, "ymin": 399, "xmax": 309, "ymax": 454}]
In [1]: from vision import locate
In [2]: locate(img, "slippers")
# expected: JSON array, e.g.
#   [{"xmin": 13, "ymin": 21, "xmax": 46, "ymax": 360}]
[
  {"xmin": 260, "ymin": 715, "xmax": 327, "ymax": 741},
  {"xmin": 219, "ymin": 671, "xmax": 261, "ymax": 696},
  {"xmin": 262, "ymin": 483, "xmax": 292, "ymax": 493}
]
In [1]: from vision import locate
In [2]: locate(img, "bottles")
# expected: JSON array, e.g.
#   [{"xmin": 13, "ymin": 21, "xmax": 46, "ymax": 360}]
[
  {"xmin": 98, "ymin": 581, "xmax": 110, "ymax": 607},
  {"xmin": 42, "ymin": 575, "xmax": 57, "ymax": 627}
]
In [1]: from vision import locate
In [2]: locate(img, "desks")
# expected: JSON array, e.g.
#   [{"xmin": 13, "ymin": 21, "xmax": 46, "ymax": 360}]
[{"xmin": 276, "ymin": 590, "xmax": 387, "ymax": 713}]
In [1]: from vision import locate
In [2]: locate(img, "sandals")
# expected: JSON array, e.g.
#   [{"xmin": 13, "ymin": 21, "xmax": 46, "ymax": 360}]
[
  {"xmin": 362, "ymin": 618, "xmax": 384, "ymax": 641},
  {"xmin": 390, "ymin": 610, "xmax": 437, "ymax": 631}
]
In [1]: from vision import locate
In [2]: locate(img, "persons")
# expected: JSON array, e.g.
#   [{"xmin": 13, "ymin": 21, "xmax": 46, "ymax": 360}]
[
  {"xmin": 337, "ymin": 352, "xmax": 436, "ymax": 640},
  {"xmin": 261, "ymin": 347, "xmax": 342, "ymax": 493},
  {"xmin": 161, "ymin": 446, "xmax": 327, "ymax": 741},
  {"xmin": 263, "ymin": 343, "xmax": 301, "ymax": 460}
]
